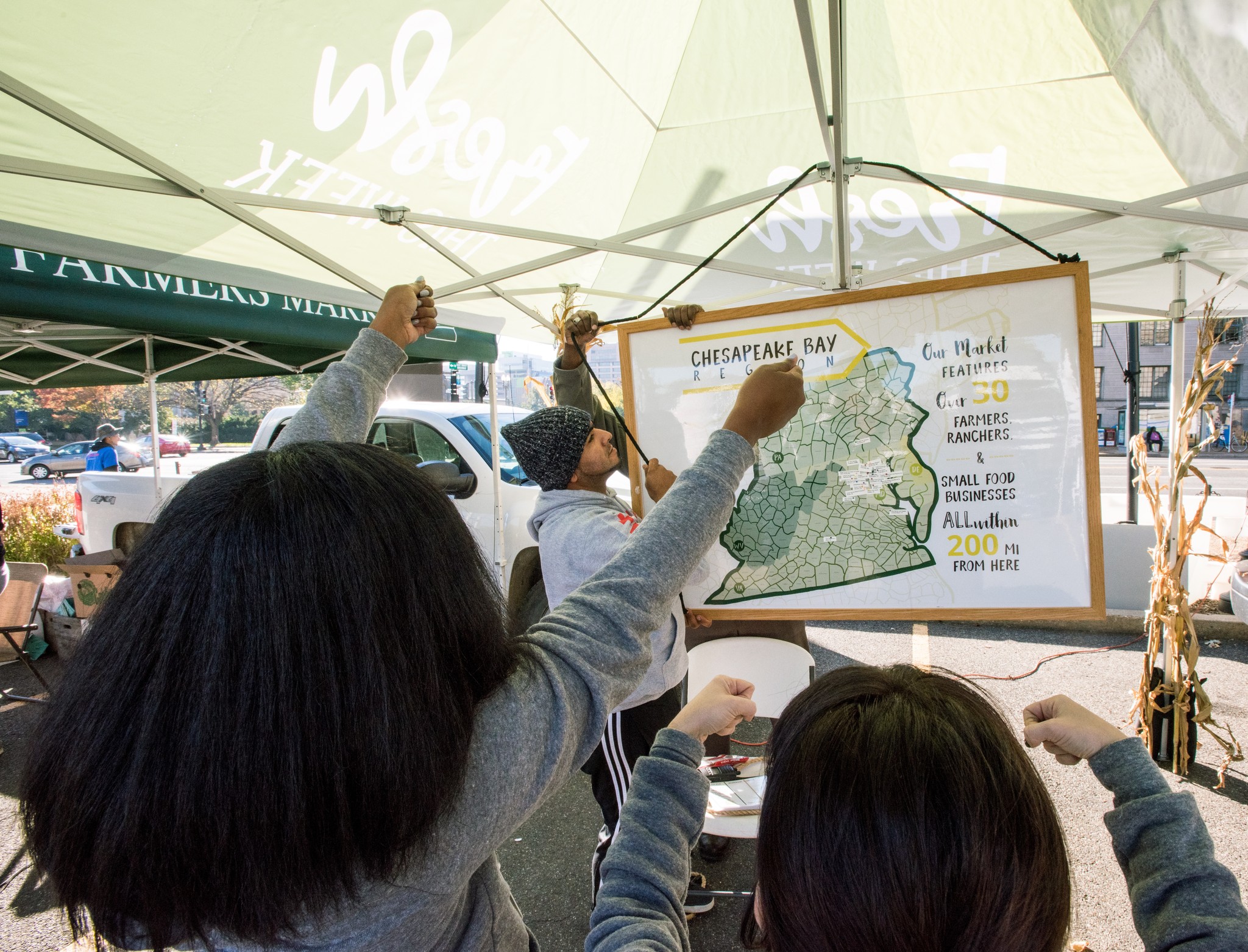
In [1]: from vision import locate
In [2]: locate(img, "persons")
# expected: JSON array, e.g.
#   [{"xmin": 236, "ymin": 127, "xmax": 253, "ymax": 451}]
[
  {"xmin": 553, "ymin": 303, "xmax": 811, "ymax": 863},
  {"xmin": 498, "ymin": 406, "xmax": 714, "ymax": 919},
  {"xmin": 16, "ymin": 275, "xmax": 807, "ymax": 952},
  {"xmin": 1146, "ymin": 426, "xmax": 1165, "ymax": 452},
  {"xmin": 86, "ymin": 424, "xmax": 124, "ymax": 472},
  {"xmin": 1213, "ymin": 417, "xmax": 1222, "ymax": 448},
  {"xmin": 584, "ymin": 662, "xmax": 1248, "ymax": 952}
]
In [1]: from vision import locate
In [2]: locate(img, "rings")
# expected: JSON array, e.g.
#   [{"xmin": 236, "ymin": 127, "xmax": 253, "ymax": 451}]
[{"xmin": 573, "ymin": 317, "xmax": 582, "ymax": 326}]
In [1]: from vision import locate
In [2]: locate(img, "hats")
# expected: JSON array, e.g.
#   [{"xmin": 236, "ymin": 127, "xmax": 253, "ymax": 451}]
[
  {"xmin": 500, "ymin": 405, "xmax": 591, "ymax": 492},
  {"xmin": 93, "ymin": 423, "xmax": 124, "ymax": 442}
]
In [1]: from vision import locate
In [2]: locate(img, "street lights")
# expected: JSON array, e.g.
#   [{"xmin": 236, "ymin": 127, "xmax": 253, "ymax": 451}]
[{"xmin": 501, "ymin": 374, "xmax": 510, "ymax": 406}]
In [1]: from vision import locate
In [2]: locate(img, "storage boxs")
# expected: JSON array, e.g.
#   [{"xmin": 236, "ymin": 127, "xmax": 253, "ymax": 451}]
[
  {"xmin": 56, "ymin": 549, "xmax": 124, "ymax": 618},
  {"xmin": 53, "ymin": 615, "xmax": 81, "ymax": 661}
]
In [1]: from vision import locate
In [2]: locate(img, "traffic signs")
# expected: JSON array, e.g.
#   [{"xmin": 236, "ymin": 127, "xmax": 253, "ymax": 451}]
[{"xmin": 449, "ymin": 362, "xmax": 468, "ymax": 370}]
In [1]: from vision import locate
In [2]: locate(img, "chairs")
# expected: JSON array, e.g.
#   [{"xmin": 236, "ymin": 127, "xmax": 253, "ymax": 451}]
[
  {"xmin": 0, "ymin": 562, "xmax": 53, "ymax": 704},
  {"xmin": 688, "ymin": 637, "xmax": 816, "ymax": 839}
]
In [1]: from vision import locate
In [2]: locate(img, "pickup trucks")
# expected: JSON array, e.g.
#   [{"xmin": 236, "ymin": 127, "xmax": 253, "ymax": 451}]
[{"xmin": 76, "ymin": 400, "xmax": 632, "ymax": 638}]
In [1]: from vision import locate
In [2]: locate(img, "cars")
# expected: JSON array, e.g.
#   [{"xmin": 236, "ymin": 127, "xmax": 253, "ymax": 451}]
[
  {"xmin": 131, "ymin": 432, "xmax": 190, "ymax": 458},
  {"xmin": 0, "ymin": 432, "xmax": 53, "ymax": 463},
  {"xmin": 20, "ymin": 440, "xmax": 153, "ymax": 480}
]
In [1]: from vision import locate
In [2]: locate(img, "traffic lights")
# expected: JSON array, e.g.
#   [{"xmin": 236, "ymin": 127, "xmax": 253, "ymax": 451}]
[
  {"xmin": 1228, "ymin": 395, "xmax": 1231, "ymax": 406},
  {"xmin": 200, "ymin": 389, "xmax": 206, "ymax": 403},
  {"xmin": 550, "ymin": 386, "xmax": 555, "ymax": 400},
  {"xmin": 463, "ymin": 383, "xmax": 469, "ymax": 398},
  {"xmin": 204, "ymin": 405, "xmax": 211, "ymax": 414}
]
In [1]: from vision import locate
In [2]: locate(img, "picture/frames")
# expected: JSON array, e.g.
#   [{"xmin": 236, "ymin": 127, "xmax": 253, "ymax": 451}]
[{"xmin": 616, "ymin": 261, "xmax": 1106, "ymax": 621}]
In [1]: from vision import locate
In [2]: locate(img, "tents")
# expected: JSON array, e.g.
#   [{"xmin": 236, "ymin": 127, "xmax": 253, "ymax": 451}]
[{"xmin": 1, "ymin": 0, "xmax": 1248, "ymax": 952}]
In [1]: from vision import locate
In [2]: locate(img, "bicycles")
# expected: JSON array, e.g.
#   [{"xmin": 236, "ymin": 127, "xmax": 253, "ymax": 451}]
[{"xmin": 1210, "ymin": 431, "xmax": 1247, "ymax": 453}]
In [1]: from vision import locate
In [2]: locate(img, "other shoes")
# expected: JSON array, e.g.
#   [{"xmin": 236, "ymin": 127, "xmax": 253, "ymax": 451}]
[{"xmin": 682, "ymin": 882, "xmax": 715, "ymax": 913}]
